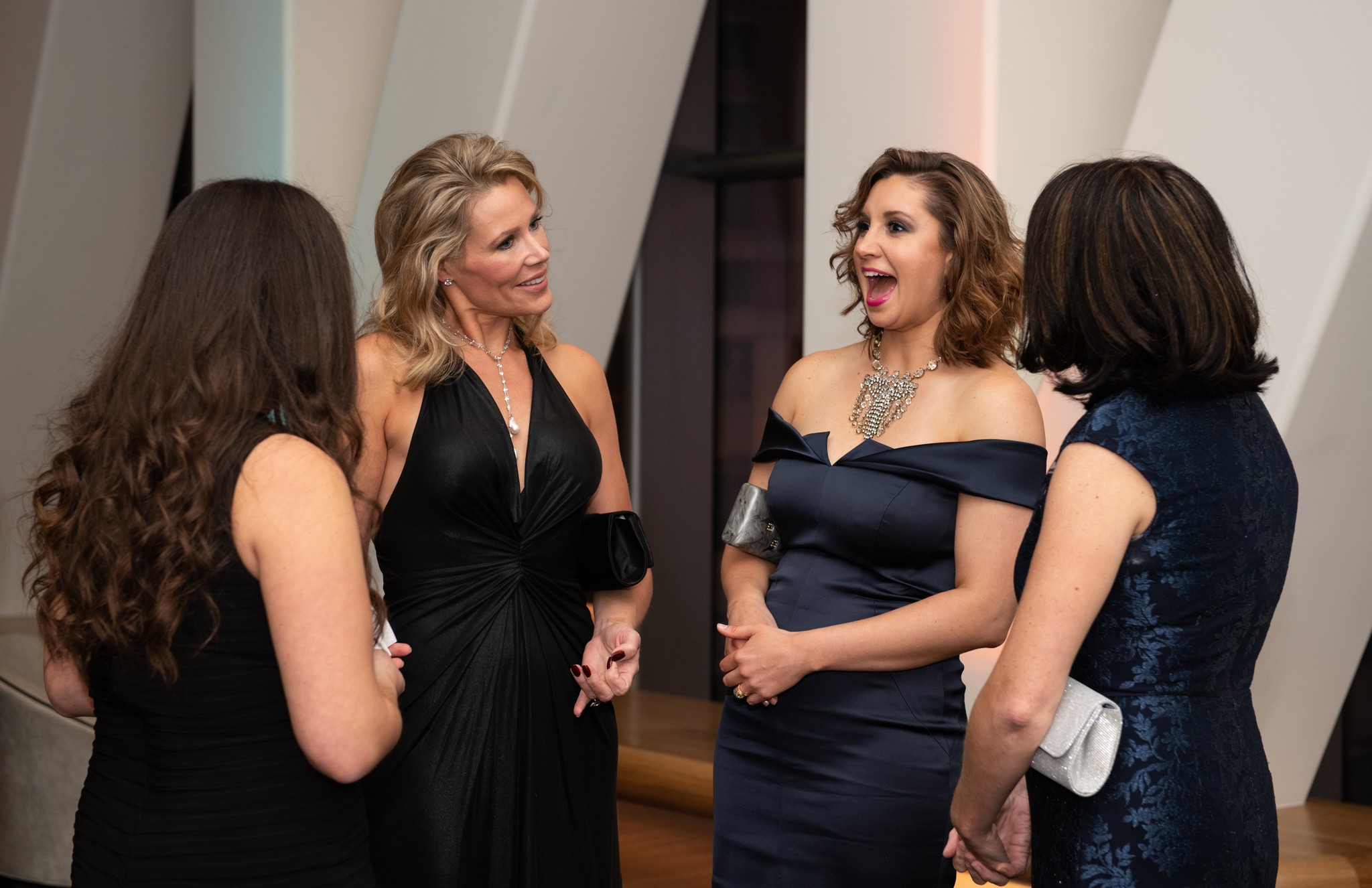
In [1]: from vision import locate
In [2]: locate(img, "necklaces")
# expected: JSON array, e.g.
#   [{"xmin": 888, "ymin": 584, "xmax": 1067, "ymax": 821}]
[
  {"xmin": 442, "ymin": 319, "xmax": 519, "ymax": 457},
  {"xmin": 849, "ymin": 329, "xmax": 944, "ymax": 439}
]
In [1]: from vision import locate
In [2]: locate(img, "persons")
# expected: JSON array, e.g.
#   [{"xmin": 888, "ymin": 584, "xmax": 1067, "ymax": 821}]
[
  {"xmin": 943, "ymin": 156, "xmax": 1298, "ymax": 888},
  {"xmin": 18, "ymin": 133, "xmax": 655, "ymax": 888},
  {"xmin": 711, "ymin": 148, "xmax": 1047, "ymax": 888}
]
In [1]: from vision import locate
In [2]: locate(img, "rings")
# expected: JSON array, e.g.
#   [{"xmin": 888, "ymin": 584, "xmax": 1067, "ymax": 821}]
[
  {"xmin": 736, "ymin": 684, "xmax": 750, "ymax": 698},
  {"xmin": 590, "ymin": 697, "xmax": 601, "ymax": 707}
]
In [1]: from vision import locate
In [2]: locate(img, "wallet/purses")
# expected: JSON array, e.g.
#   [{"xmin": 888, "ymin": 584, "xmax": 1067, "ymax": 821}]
[
  {"xmin": 721, "ymin": 483, "xmax": 783, "ymax": 566},
  {"xmin": 580, "ymin": 510, "xmax": 654, "ymax": 593},
  {"xmin": 1029, "ymin": 676, "xmax": 1123, "ymax": 798}
]
